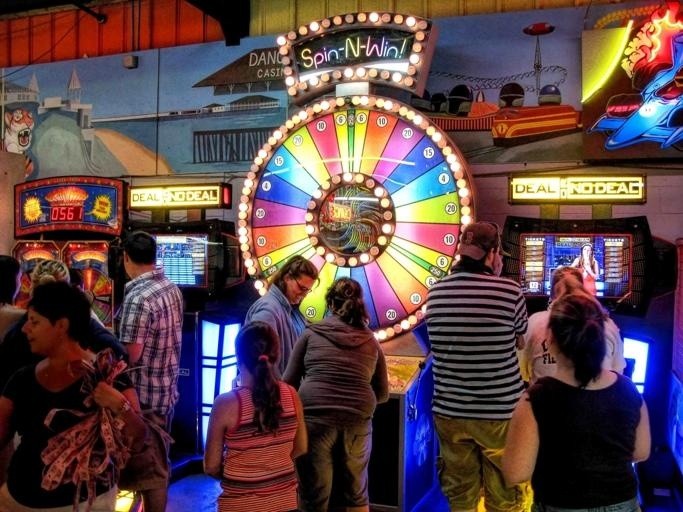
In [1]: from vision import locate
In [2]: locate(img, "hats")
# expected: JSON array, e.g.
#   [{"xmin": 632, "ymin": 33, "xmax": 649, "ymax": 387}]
[{"xmin": 459, "ymin": 221, "xmax": 501, "ymax": 261}]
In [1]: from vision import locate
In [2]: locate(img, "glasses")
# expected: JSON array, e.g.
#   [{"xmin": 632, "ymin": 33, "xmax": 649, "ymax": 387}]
[{"xmin": 293, "ymin": 276, "xmax": 312, "ymax": 294}]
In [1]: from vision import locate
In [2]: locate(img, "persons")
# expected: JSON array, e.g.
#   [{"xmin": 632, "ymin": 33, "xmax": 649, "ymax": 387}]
[
  {"xmin": 203, "ymin": 321, "xmax": 307, "ymax": 512},
  {"xmin": 0, "ymin": 260, "xmax": 124, "ymax": 375},
  {"xmin": 572, "ymin": 243, "xmax": 599, "ymax": 295},
  {"xmin": 423, "ymin": 222, "xmax": 533, "ymax": 512},
  {"xmin": 0, "ymin": 282, "xmax": 143, "ymax": 511},
  {"xmin": 0, "ymin": 254, "xmax": 27, "ymax": 342},
  {"xmin": 118, "ymin": 232, "xmax": 184, "ymax": 512},
  {"xmin": 520, "ymin": 267, "xmax": 627, "ymax": 380},
  {"xmin": 245, "ymin": 254, "xmax": 320, "ymax": 378},
  {"xmin": 280, "ymin": 276, "xmax": 389, "ymax": 512},
  {"xmin": 500, "ymin": 294, "xmax": 651, "ymax": 512}
]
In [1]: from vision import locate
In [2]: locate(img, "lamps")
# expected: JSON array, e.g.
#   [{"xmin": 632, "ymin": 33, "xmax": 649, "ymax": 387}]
[{"xmin": 199, "ymin": 313, "xmax": 242, "ymax": 456}]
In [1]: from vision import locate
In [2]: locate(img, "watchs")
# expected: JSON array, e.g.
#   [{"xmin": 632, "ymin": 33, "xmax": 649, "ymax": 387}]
[{"xmin": 119, "ymin": 400, "xmax": 131, "ymax": 414}]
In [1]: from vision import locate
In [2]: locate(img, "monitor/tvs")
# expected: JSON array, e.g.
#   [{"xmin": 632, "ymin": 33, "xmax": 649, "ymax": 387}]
[
  {"xmin": 151, "ymin": 233, "xmax": 209, "ymax": 290},
  {"xmin": 519, "ymin": 232, "xmax": 634, "ymax": 303}
]
